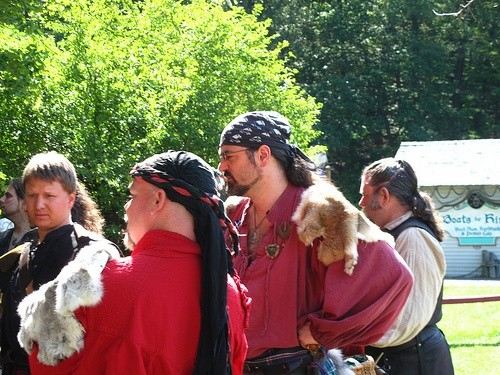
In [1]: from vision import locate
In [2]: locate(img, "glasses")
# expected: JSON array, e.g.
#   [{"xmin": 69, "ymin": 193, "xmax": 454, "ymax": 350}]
[{"xmin": 219, "ymin": 148, "xmax": 251, "ymax": 161}]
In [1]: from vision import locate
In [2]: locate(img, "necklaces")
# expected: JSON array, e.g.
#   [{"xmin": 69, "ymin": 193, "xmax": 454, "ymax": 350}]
[{"xmin": 249, "ymin": 206, "xmax": 266, "ymax": 244}]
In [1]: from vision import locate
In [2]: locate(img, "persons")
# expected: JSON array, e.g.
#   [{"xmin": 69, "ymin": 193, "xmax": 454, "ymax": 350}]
[
  {"xmin": 0, "ymin": 111, "xmax": 414, "ymax": 375},
  {"xmin": 358, "ymin": 156, "xmax": 455, "ymax": 375}
]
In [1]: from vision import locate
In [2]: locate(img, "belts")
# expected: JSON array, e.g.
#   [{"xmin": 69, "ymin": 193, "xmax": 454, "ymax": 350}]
[
  {"xmin": 249, "ymin": 350, "xmax": 329, "ymax": 375},
  {"xmin": 380, "ymin": 325, "xmax": 438, "ymax": 352}
]
